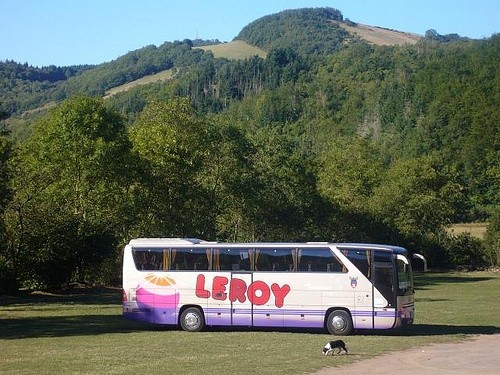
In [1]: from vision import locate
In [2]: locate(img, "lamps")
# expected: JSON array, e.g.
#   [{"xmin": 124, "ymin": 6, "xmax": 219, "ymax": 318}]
[
  {"xmin": 141, "ymin": 262, "xmax": 152, "ymax": 270},
  {"xmin": 308, "ymin": 264, "xmax": 312, "ymax": 272},
  {"xmin": 326, "ymin": 264, "xmax": 331, "ymax": 272},
  {"xmin": 193, "ymin": 263, "xmax": 198, "ymax": 269},
  {"xmin": 272, "ymin": 264, "xmax": 276, "ymax": 272},
  {"xmin": 175, "ymin": 263, "xmax": 180, "ymax": 270}
]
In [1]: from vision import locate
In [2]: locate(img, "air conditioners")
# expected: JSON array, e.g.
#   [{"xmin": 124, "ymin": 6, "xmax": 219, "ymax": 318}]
[{"xmin": 322, "ymin": 340, "xmax": 348, "ymax": 356}]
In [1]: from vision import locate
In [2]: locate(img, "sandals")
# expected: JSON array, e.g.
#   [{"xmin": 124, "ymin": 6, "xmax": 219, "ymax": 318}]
[{"xmin": 120, "ymin": 235, "xmax": 429, "ymax": 338}]
